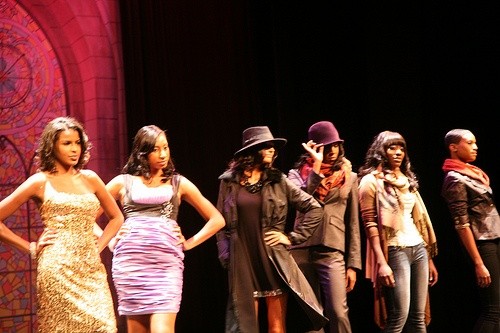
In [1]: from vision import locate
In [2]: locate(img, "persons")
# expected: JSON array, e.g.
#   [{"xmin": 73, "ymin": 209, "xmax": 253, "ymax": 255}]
[
  {"xmin": 215, "ymin": 125, "xmax": 324, "ymax": 333},
  {"xmin": 1, "ymin": 115, "xmax": 125, "ymax": 333},
  {"xmin": 357, "ymin": 131, "xmax": 439, "ymax": 333},
  {"xmin": 90, "ymin": 124, "xmax": 227, "ymax": 333},
  {"xmin": 444, "ymin": 128, "xmax": 499, "ymax": 333},
  {"xmin": 287, "ymin": 121, "xmax": 362, "ymax": 332}
]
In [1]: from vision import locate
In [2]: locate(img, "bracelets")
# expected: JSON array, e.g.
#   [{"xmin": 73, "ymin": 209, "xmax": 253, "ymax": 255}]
[{"xmin": 28, "ymin": 241, "xmax": 38, "ymax": 260}]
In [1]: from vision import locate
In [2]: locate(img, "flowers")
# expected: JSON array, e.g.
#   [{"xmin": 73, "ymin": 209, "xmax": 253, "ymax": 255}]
[{"xmin": 160, "ymin": 201, "xmax": 174, "ymax": 219}]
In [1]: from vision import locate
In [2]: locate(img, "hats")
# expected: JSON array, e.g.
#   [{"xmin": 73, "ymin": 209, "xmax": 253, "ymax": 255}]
[
  {"xmin": 308, "ymin": 120, "xmax": 345, "ymax": 145},
  {"xmin": 234, "ymin": 125, "xmax": 287, "ymax": 155}
]
